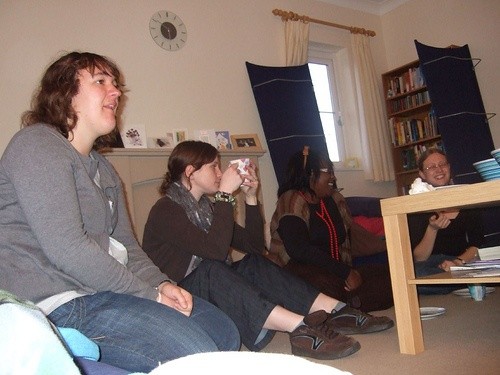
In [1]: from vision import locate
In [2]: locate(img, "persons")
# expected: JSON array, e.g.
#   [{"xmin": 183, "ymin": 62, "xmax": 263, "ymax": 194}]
[
  {"xmin": 268, "ymin": 146, "xmax": 395, "ymax": 313},
  {"xmin": 406, "ymin": 149, "xmax": 483, "ymax": 296},
  {"xmin": 142, "ymin": 140, "xmax": 394, "ymax": 360}
]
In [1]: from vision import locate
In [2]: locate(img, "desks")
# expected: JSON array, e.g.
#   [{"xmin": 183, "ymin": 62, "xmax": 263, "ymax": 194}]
[
  {"xmin": 98, "ymin": 147, "xmax": 267, "ymax": 265},
  {"xmin": 378, "ymin": 178, "xmax": 500, "ymax": 356}
]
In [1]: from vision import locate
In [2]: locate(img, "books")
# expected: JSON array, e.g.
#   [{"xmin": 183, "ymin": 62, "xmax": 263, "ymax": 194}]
[{"xmin": 386, "ymin": 67, "xmax": 445, "ymax": 170}]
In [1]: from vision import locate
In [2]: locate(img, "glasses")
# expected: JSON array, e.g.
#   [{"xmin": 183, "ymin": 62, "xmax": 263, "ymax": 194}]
[
  {"xmin": 423, "ymin": 163, "xmax": 449, "ymax": 171},
  {"xmin": 319, "ymin": 168, "xmax": 334, "ymax": 174}
]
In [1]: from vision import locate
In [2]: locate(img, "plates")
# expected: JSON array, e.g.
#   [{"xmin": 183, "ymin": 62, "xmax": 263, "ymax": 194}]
[
  {"xmin": 453, "ymin": 287, "xmax": 496, "ymax": 297},
  {"xmin": 420, "ymin": 307, "xmax": 446, "ymax": 320}
]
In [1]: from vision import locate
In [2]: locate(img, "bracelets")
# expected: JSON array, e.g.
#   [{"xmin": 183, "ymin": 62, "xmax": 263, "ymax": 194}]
[
  {"xmin": 456, "ymin": 256, "xmax": 465, "ymax": 265},
  {"xmin": 215, "ymin": 191, "xmax": 235, "ymax": 206},
  {"xmin": 158, "ymin": 293, "xmax": 161, "ymax": 303}
]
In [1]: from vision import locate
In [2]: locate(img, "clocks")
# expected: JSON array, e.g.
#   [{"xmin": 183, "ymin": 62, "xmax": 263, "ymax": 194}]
[{"xmin": 148, "ymin": 8, "xmax": 188, "ymax": 52}]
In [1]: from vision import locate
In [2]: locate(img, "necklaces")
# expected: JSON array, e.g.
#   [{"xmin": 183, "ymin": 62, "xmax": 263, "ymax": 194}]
[
  {"xmin": 297, "ymin": 191, "xmax": 338, "ymax": 261},
  {"xmin": 0, "ymin": 51, "xmax": 241, "ymax": 375}
]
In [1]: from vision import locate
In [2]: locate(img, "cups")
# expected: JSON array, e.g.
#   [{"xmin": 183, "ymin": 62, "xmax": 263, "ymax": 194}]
[
  {"xmin": 468, "ymin": 286, "xmax": 486, "ymax": 301},
  {"xmin": 227, "ymin": 158, "xmax": 251, "ymax": 188}
]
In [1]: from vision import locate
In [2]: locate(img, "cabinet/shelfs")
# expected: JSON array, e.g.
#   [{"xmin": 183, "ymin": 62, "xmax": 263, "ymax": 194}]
[{"xmin": 379, "ymin": 42, "xmax": 462, "ymax": 197}]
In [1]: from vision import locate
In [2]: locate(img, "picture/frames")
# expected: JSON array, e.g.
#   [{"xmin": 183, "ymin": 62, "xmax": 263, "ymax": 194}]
[
  {"xmin": 230, "ymin": 132, "xmax": 263, "ymax": 151},
  {"xmin": 172, "ymin": 127, "xmax": 188, "ymax": 146}
]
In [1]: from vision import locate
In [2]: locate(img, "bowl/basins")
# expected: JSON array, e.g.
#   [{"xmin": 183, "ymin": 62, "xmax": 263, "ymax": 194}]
[
  {"xmin": 490, "ymin": 148, "xmax": 500, "ymax": 166},
  {"xmin": 472, "ymin": 158, "xmax": 500, "ymax": 181}
]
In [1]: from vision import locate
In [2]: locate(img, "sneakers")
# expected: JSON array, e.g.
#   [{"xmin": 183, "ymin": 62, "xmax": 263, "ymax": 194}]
[
  {"xmin": 289, "ymin": 310, "xmax": 361, "ymax": 360},
  {"xmin": 323, "ymin": 302, "xmax": 394, "ymax": 335}
]
